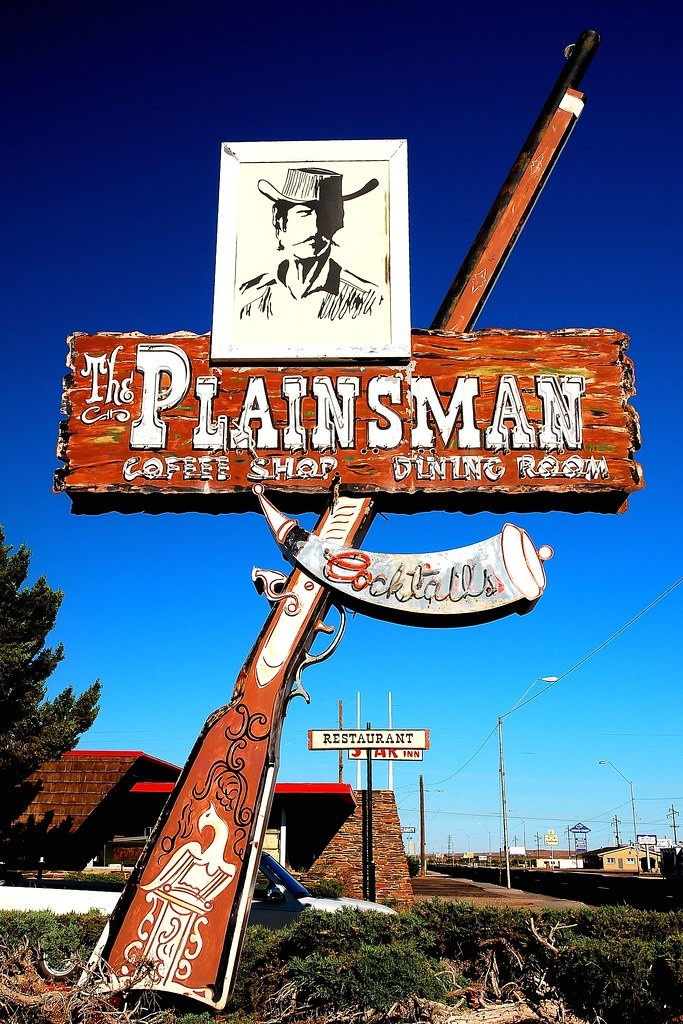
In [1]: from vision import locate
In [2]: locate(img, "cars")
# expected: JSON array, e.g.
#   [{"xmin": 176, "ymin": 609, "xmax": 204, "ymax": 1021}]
[{"xmin": 240, "ymin": 848, "xmax": 401, "ymax": 940}]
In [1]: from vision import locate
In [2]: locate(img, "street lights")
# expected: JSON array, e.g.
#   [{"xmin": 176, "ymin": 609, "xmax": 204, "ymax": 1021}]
[
  {"xmin": 496, "ymin": 676, "xmax": 560, "ymax": 889},
  {"xmin": 598, "ymin": 760, "xmax": 639, "ymax": 847},
  {"xmin": 418, "ymin": 788, "xmax": 444, "ymax": 878},
  {"xmin": 509, "ymin": 809, "xmax": 526, "ymax": 848},
  {"xmin": 479, "ymin": 822, "xmax": 492, "ymax": 851},
  {"xmin": 458, "ymin": 828, "xmax": 472, "ymax": 854}
]
